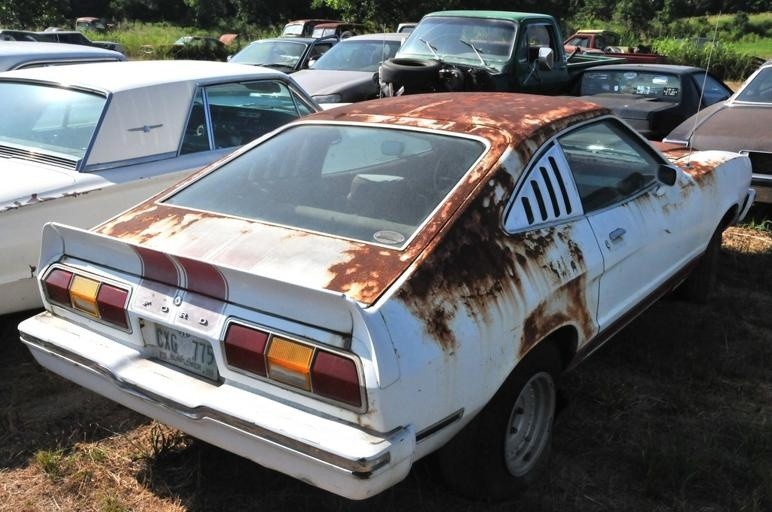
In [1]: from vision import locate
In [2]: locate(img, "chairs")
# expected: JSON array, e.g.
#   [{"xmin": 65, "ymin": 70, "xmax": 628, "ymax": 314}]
[{"xmin": 348, "ymin": 172, "xmax": 424, "ymax": 229}]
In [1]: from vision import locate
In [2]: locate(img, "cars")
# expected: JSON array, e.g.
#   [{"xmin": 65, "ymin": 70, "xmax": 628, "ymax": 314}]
[{"xmin": 16, "ymin": 87, "xmax": 761, "ymax": 503}]
